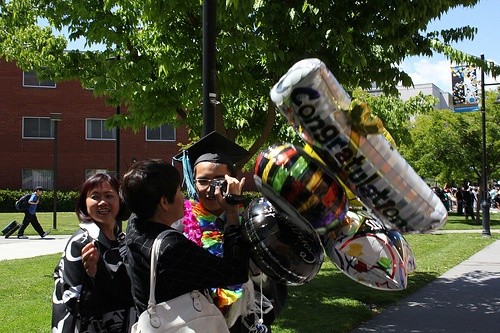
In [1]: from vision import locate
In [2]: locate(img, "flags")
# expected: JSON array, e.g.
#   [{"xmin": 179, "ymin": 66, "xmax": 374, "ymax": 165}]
[{"xmin": 451, "ymin": 66, "xmax": 479, "ymax": 112}]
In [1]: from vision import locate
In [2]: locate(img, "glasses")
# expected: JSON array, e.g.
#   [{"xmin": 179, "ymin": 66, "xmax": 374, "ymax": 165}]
[{"xmin": 195, "ymin": 179, "xmax": 210, "ymax": 187}]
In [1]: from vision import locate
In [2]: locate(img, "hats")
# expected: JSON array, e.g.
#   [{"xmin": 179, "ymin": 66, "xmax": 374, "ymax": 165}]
[{"xmin": 172, "ymin": 130, "xmax": 254, "ymax": 199}]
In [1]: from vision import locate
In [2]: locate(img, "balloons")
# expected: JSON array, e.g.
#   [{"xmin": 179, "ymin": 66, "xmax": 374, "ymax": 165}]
[
  {"xmin": 300, "ymin": 105, "xmax": 398, "ymax": 208},
  {"xmin": 269, "ymin": 58, "xmax": 448, "ymax": 235},
  {"xmin": 320, "ymin": 210, "xmax": 416, "ymax": 291},
  {"xmin": 242, "ymin": 195, "xmax": 325, "ymax": 287},
  {"xmin": 254, "ymin": 141, "xmax": 350, "ymax": 233}
]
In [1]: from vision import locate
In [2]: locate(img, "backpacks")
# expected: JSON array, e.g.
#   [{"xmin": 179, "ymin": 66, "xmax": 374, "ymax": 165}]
[{"xmin": 15, "ymin": 193, "xmax": 32, "ymax": 211}]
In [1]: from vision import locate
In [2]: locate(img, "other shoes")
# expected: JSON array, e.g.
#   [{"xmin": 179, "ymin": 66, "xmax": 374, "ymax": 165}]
[
  {"xmin": 18, "ymin": 235, "xmax": 28, "ymax": 239},
  {"xmin": 42, "ymin": 230, "xmax": 51, "ymax": 237}
]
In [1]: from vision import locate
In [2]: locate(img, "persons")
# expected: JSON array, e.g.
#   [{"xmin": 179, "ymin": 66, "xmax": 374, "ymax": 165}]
[
  {"xmin": 51, "ymin": 174, "xmax": 142, "ymax": 333},
  {"xmin": 16, "ymin": 186, "xmax": 51, "ymax": 239},
  {"xmin": 426, "ymin": 177, "xmax": 500, "ymax": 221},
  {"xmin": 169, "ymin": 130, "xmax": 289, "ymax": 333},
  {"xmin": 121, "ymin": 158, "xmax": 252, "ymax": 333}
]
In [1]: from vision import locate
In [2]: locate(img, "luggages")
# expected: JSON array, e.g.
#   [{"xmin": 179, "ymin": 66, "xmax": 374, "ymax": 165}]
[{"xmin": 1, "ymin": 219, "xmax": 25, "ymax": 238}]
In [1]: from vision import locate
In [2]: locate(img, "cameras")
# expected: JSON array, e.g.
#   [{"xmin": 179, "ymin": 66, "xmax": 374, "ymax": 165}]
[{"xmin": 208, "ymin": 179, "xmax": 228, "ymax": 199}]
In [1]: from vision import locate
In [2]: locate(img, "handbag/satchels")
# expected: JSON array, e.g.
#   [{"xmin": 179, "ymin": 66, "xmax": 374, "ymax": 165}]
[{"xmin": 130, "ymin": 228, "xmax": 229, "ymax": 333}]
